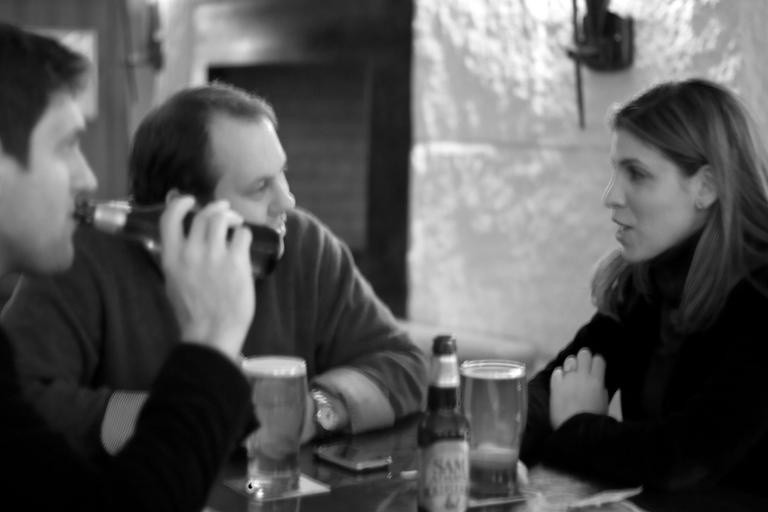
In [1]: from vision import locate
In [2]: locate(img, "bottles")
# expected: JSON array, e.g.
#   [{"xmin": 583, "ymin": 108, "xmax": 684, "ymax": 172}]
[
  {"xmin": 416, "ymin": 335, "xmax": 473, "ymax": 512},
  {"xmin": 72, "ymin": 196, "xmax": 283, "ymax": 284}
]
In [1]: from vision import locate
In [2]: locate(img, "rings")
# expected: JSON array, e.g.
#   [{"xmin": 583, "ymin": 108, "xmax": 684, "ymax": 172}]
[{"xmin": 563, "ymin": 366, "xmax": 579, "ymax": 376}]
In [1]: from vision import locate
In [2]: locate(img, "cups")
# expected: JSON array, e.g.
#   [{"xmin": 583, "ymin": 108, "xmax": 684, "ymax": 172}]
[
  {"xmin": 460, "ymin": 357, "xmax": 529, "ymax": 502},
  {"xmin": 241, "ymin": 355, "xmax": 309, "ymax": 498}
]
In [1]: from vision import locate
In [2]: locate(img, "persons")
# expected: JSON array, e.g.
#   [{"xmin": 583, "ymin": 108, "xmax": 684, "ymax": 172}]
[
  {"xmin": 518, "ymin": 77, "xmax": 767, "ymax": 511},
  {"xmin": 0, "ymin": 23, "xmax": 256, "ymax": 510},
  {"xmin": 2, "ymin": 87, "xmax": 432, "ymax": 463}
]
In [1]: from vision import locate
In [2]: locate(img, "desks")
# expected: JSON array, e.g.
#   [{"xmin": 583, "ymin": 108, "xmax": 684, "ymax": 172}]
[{"xmin": 203, "ymin": 410, "xmax": 643, "ymax": 511}]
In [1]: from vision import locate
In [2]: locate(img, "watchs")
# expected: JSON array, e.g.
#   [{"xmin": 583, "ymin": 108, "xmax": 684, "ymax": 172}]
[{"xmin": 310, "ymin": 387, "xmax": 340, "ymax": 438}]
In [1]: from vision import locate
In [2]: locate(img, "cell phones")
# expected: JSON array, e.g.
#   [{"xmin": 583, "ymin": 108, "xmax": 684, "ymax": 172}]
[{"xmin": 316, "ymin": 439, "xmax": 393, "ymax": 474}]
